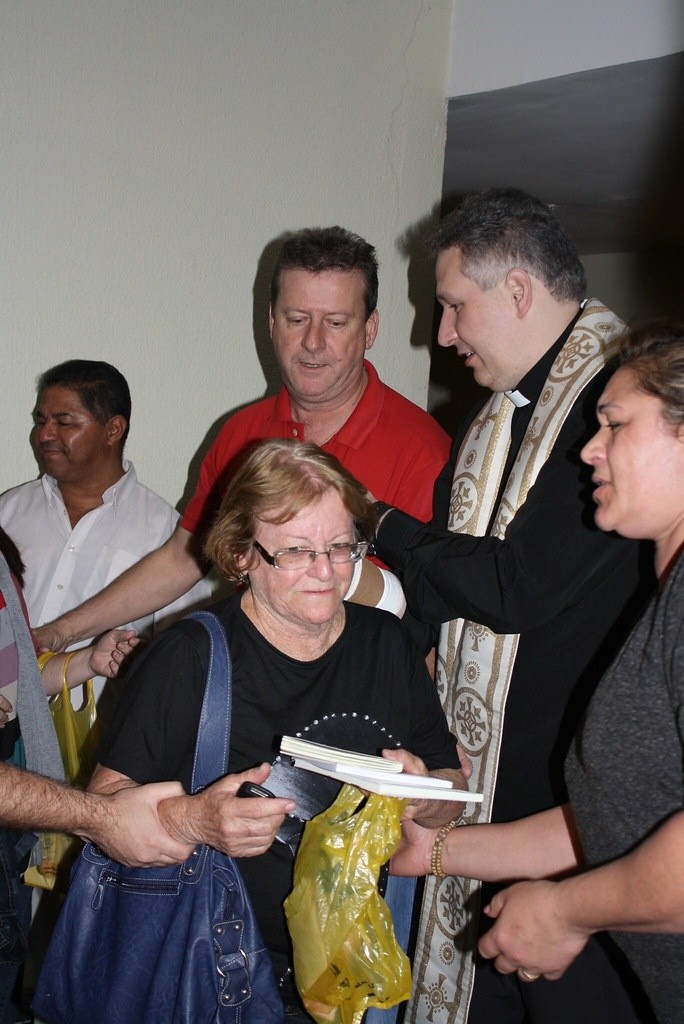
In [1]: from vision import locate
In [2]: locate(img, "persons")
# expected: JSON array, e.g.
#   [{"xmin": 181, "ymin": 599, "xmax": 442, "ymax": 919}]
[
  {"xmin": 0, "ymin": 359, "xmax": 234, "ymax": 738},
  {"xmin": 358, "ymin": 186, "xmax": 658, "ymax": 1024},
  {"xmin": 0, "ymin": 525, "xmax": 145, "ymax": 1024},
  {"xmin": 0, "ymin": 762, "xmax": 197, "ymax": 867},
  {"xmin": 388, "ymin": 337, "xmax": 684, "ymax": 1024},
  {"xmin": 31, "ymin": 225, "xmax": 453, "ymax": 680},
  {"xmin": 77, "ymin": 442, "xmax": 470, "ymax": 1024}
]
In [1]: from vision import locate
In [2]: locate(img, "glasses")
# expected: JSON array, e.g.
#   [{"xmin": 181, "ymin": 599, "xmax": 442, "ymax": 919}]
[{"xmin": 252, "ymin": 524, "xmax": 370, "ymax": 570}]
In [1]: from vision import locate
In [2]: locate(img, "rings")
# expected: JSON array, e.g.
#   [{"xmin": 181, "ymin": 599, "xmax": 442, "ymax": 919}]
[{"xmin": 522, "ymin": 969, "xmax": 539, "ymax": 980}]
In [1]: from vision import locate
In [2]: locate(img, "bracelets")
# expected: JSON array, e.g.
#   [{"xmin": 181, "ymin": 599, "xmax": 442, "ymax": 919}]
[{"xmin": 432, "ymin": 820, "xmax": 456, "ymax": 878}]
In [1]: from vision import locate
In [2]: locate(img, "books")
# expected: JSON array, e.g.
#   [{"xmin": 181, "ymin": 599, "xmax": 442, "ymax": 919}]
[{"xmin": 280, "ymin": 736, "xmax": 484, "ymax": 802}]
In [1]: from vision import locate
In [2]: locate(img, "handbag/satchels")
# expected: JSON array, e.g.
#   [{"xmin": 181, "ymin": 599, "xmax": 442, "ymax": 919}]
[
  {"xmin": 25, "ymin": 651, "xmax": 96, "ymax": 892},
  {"xmin": 34, "ymin": 608, "xmax": 285, "ymax": 1024}
]
